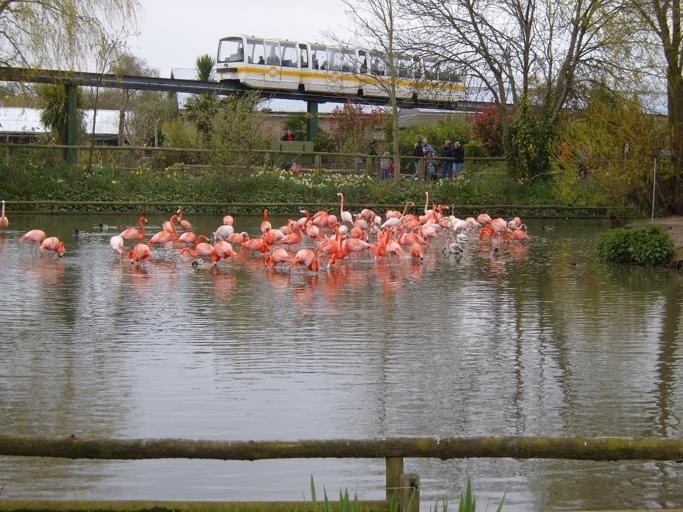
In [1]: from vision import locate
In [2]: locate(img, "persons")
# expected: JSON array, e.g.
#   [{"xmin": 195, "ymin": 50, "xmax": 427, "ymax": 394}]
[
  {"xmin": 367, "ymin": 138, "xmax": 464, "ymax": 182},
  {"xmin": 279, "ymin": 159, "xmax": 303, "ymax": 182},
  {"xmin": 237, "ymin": 47, "xmax": 458, "ymax": 82},
  {"xmin": 280, "ymin": 127, "xmax": 295, "ymax": 141}
]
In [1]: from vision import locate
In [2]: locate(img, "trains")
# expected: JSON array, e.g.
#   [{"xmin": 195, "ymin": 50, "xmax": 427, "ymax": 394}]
[{"xmin": 215, "ymin": 33, "xmax": 467, "ymax": 102}]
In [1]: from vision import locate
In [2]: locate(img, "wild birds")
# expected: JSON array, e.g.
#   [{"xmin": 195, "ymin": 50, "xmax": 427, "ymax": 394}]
[
  {"xmin": 18, "ymin": 230, "xmax": 46, "ymax": 254},
  {"xmin": 110, "ymin": 191, "xmax": 533, "ymax": 277},
  {"xmin": 40, "ymin": 236, "xmax": 65, "ymax": 257},
  {"xmin": 0, "ymin": 200, "xmax": 9, "ymax": 235}
]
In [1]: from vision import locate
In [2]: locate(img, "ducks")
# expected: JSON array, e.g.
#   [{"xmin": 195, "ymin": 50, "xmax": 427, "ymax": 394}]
[
  {"xmin": 99, "ymin": 224, "xmax": 110, "ymax": 228},
  {"xmin": 73, "ymin": 229, "xmax": 89, "ymax": 234}
]
